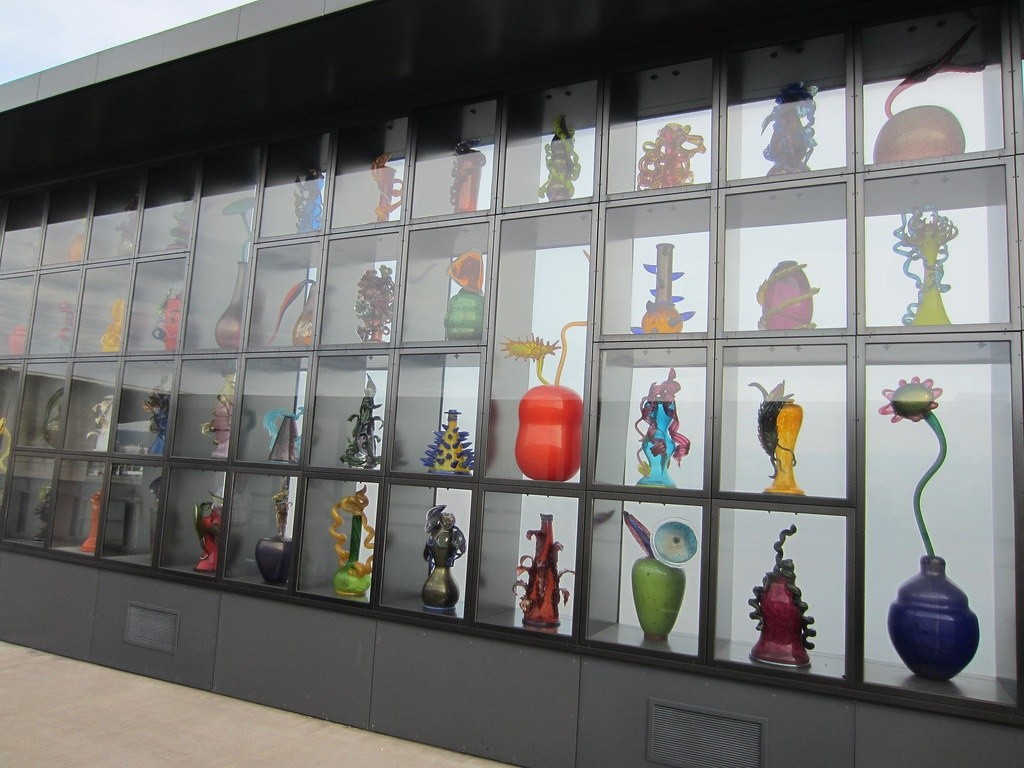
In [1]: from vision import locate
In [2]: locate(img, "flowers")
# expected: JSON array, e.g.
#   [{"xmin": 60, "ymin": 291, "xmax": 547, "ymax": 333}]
[
  {"xmin": 501, "ymin": 249, "xmax": 590, "ymax": 385},
  {"xmin": 877, "ymin": 376, "xmax": 949, "ymax": 559}
]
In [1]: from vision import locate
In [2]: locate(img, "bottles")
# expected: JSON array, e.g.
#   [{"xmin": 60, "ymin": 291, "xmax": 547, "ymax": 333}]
[{"xmin": 216, "ymin": 261, "xmax": 243, "ymax": 348}]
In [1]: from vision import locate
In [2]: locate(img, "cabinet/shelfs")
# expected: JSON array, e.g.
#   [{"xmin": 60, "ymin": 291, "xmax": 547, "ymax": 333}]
[{"xmin": 0, "ymin": 0, "xmax": 1024, "ymax": 768}]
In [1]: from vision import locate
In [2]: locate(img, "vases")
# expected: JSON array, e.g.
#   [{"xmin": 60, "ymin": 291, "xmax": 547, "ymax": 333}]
[
  {"xmin": 632, "ymin": 556, "xmax": 685, "ymax": 641},
  {"xmin": 873, "ymin": 24, "xmax": 987, "ymax": 163},
  {"xmin": 887, "ymin": 556, "xmax": 979, "ymax": 683},
  {"xmin": 636, "ymin": 121, "xmax": 707, "ymax": 190},
  {"xmin": 514, "ymin": 514, "xmax": 577, "ymax": 629},
  {"xmin": 538, "ymin": 113, "xmax": 581, "ymax": 199},
  {"xmin": 759, "ymin": 80, "xmax": 819, "ymax": 173},
  {"xmin": 634, "ymin": 366, "xmax": 690, "ymax": 488},
  {"xmin": 629, "ymin": 242, "xmax": 696, "ymax": 332},
  {"xmin": 0, "ymin": 136, "xmax": 486, "ymax": 615},
  {"xmin": 893, "ymin": 204, "xmax": 959, "ymax": 325},
  {"xmin": 749, "ymin": 525, "xmax": 818, "ymax": 669},
  {"xmin": 756, "ymin": 260, "xmax": 820, "ymax": 330},
  {"xmin": 747, "ymin": 378, "xmax": 805, "ymax": 497},
  {"xmin": 514, "ymin": 383, "xmax": 583, "ymax": 483}
]
